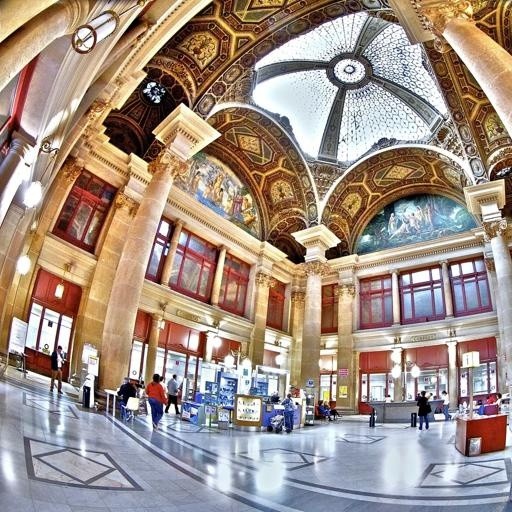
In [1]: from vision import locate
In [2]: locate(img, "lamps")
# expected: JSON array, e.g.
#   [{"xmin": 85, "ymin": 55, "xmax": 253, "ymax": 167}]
[
  {"xmin": 53, "ymin": 261, "xmax": 74, "ymax": 301},
  {"xmin": 22, "ymin": 139, "xmax": 60, "ymax": 212},
  {"xmin": 15, "ymin": 228, "xmax": 35, "ymax": 277},
  {"xmin": 69, "ymin": 0, "xmax": 154, "ymax": 56}
]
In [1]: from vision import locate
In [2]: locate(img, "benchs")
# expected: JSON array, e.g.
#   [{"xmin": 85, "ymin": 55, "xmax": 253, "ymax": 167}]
[{"xmin": 314, "ymin": 404, "xmax": 325, "ymax": 422}]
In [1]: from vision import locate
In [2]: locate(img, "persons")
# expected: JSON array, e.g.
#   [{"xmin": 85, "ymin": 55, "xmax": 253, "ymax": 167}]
[
  {"xmin": 117, "ymin": 377, "xmax": 136, "ymax": 421},
  {"xmin": 384, "ymin": 395, "xmax": 393, "ymax": 403},
  {"xmin": 415, "ymin": 391, "xmax": 433, "ymax": 430},
  {"xmin": 159, "ymin": 377, "xmax": 169, "ymax": 399},
  {"xmin": 146, "ymin": 374, "xmax": 168, "ymax": 428},
  {"xmin": 271, "ymin": 391, "xmax": 280, "ymax": 402},
  {"xmin": 165, "ymin": 375, "xmax": 180, "ymax": 414},
  {"xmin": 190, "ymin": 171, "xmax": 248, "ymax": 215},
  {"xmin": 50, "ymin": 346, "xmax": 65, "ymax": 395},
  {"xmin": 318, "ymin": 400, "xmax": 342, "ymax": 421},
  {"xmin": 388, "ymin": 203, "xmax": 433, "ymax": 235},
  {"xmin": 442, "ymin": 391, "xmax": 452, "ymax": 421},
  {"xmin": 494, "ymin": 394, "xmax": 504, "ymax": 411},
  {"xmin": 281, "ymin": 394, "xmax": 295, "ymax": 431}
]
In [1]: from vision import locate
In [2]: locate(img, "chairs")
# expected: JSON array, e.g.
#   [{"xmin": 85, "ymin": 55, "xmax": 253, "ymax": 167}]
[{"xmin": 121, "ymin": 397, "xmax": 140, "ymax": 424}]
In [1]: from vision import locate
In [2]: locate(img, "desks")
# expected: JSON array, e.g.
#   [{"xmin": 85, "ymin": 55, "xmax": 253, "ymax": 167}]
[
  {"xmin": 456, "ymin": 413, "xmax": 508, "ymax": 458},
  {"xmin": 103, "ymin": 388, "xmax": 117, "ymax": 419}
]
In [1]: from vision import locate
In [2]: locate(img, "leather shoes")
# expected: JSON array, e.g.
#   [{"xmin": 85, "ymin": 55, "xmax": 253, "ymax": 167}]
[
  {"xmin": 58, "ymin": 390, "xmax": 62, "ymax": 394},
  {"xmin": 50, "ymin": 389, "xmax": 52, "ymax": 391}
]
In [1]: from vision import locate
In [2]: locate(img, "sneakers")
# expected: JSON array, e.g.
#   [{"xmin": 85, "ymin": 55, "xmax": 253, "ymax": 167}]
[{"xmin": 153, "ymin": 422, "xmax": 157, "ymax": 432}]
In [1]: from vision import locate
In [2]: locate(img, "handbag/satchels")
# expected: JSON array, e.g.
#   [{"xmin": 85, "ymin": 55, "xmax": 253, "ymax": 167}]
[{"xmin": 427, "ymin": 405, "xmax": 431, "ymax": 414}]
